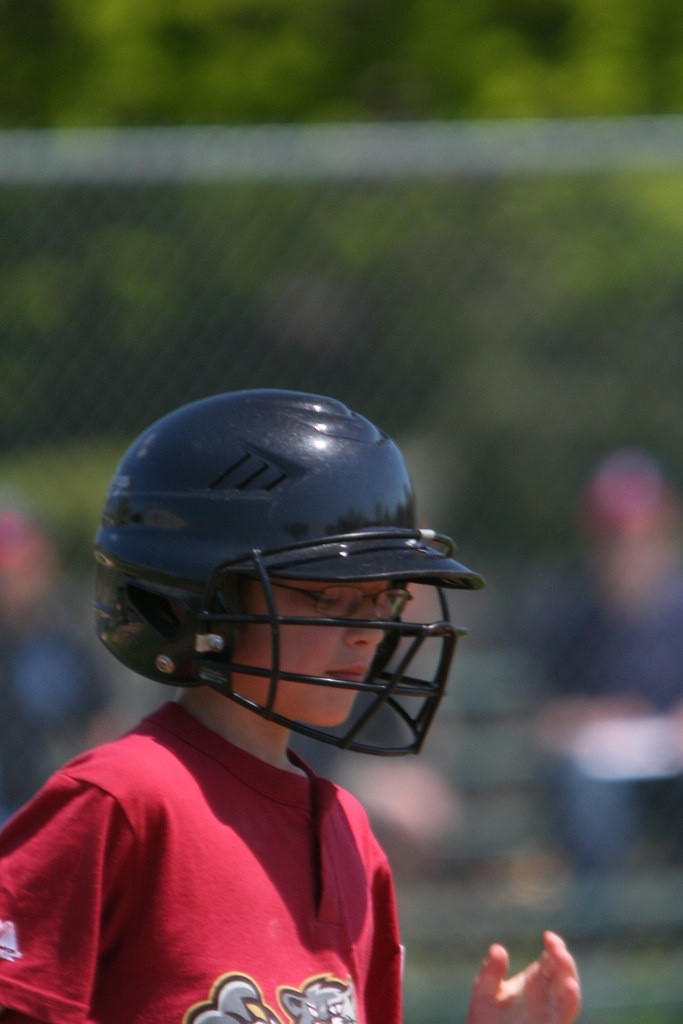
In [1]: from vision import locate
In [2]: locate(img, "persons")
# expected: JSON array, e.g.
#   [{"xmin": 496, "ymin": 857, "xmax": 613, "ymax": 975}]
[
  {"xmin": 0, "ymin": 385, "xmax": 582, "ymax": 1023},
  {"xmin": 0, "ymin": 452, "xmax": 683, "ymax": 878}
]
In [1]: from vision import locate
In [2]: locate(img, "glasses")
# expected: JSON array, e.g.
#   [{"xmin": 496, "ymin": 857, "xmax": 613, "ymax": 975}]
[{"xmin": 241, "ymin": 577, "xmax": 414, "ymax": 622}]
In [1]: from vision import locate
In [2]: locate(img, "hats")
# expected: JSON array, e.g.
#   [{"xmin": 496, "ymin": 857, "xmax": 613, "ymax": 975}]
[
  {"xmin": 590, "ymin": 457, "xmax": 672, "ymax": 537},
  {"xmin": 0, "ymin": 506, "xmax": 53, "ymax": 576}
]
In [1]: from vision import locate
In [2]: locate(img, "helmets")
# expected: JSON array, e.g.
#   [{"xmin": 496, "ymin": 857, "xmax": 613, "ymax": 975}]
[{"xmin": 96, "ymin": 388, "xmax": 487, "ymax": 758}]
